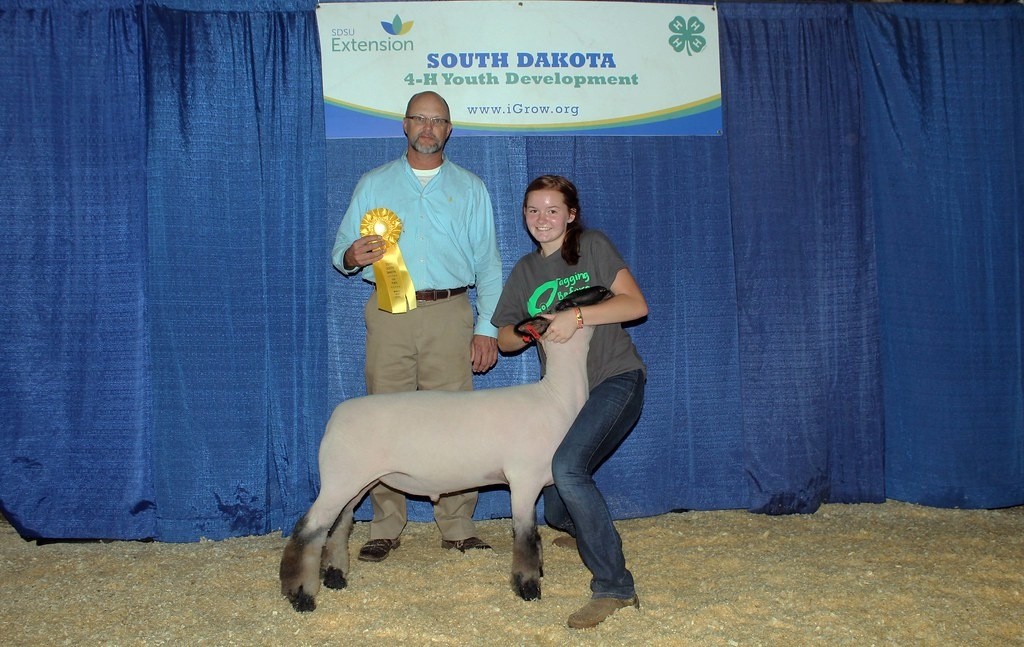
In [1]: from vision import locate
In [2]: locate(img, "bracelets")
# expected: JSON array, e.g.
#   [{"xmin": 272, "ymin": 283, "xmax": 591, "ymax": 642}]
[{"xmin": 574, "ymin": 307, "xmax": 584, "ymax": 329}]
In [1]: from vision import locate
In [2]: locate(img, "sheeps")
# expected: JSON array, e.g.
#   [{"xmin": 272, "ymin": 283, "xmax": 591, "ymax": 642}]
[{"xmin": 279, "ymin": 285, "xmax": 617, "ymax": 615}]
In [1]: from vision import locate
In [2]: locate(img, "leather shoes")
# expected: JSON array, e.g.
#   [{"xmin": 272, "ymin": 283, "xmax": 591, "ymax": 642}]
[
  {"xmin": 442, "ymin": 537, "xmax": 491, "ymax": 553},
  {"xmin": 358, "ymin": 538, "xmax": 400, "ymax": 563}
]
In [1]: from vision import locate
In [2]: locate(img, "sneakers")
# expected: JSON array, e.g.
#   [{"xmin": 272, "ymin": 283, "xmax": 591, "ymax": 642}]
[
  {"xmin": 552, "ymin": 537, "xmax": 577, "ymax": 551},
  {"xmin": 568, "ymin": 594, "xmax": 640, "ymax": 629}
]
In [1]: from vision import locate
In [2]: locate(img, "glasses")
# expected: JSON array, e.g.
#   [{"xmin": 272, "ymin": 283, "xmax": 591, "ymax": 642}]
[{"xmin": 406, "ymin": 116, "xmax": 449, "ymax": 127}]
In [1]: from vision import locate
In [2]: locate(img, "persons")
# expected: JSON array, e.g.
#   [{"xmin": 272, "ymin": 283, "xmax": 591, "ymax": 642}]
[
  {"xmin": 332, "ymin": 92, "xmax": 503, "ymax": 563},
  {"xmin": 490, "ymin": 175, "xmax": 649, "ymax": 628}
]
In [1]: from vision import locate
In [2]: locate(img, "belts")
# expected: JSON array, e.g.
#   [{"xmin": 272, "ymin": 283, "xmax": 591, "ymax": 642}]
[{"xmin": 374, "ymin": 283, "xmax": 468, "ymax": 301}]
